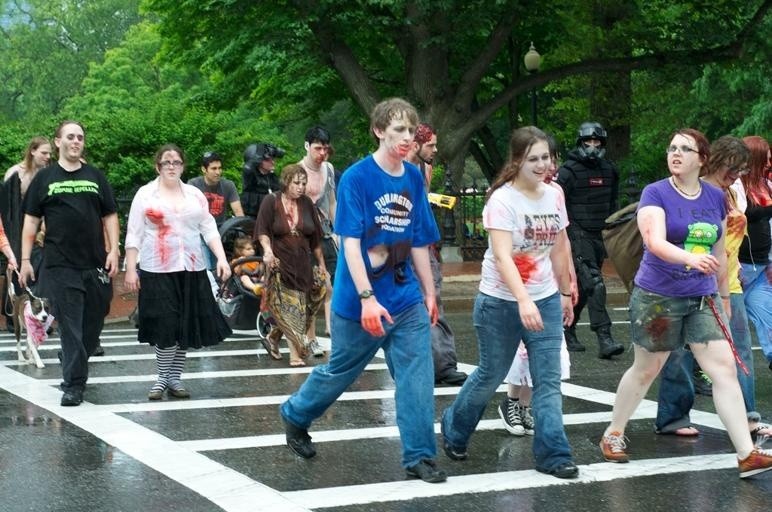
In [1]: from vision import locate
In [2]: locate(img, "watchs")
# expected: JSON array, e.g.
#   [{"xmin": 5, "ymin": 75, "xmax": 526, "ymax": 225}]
[{"xmin": 356, "ymin": 291, "xmax": 373, "ymax": 301}]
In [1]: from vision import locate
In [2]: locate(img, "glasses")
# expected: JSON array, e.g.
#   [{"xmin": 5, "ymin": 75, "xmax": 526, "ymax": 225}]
[
  {"xmin": 157, "ymin": 160, "xmax": 185, "ymax": 166},
  {"xmin": 721, "ymin": 162, "xmax": 750, "ymax": 175},
  {"xmin": 665, "ymin": 145, "xmax": 705, "ymax": 156}
]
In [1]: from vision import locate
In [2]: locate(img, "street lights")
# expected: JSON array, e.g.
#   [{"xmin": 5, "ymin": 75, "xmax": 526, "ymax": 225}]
[{"xmin": 524, "ymin": 40, "xmax": 540, "ymax": 126}]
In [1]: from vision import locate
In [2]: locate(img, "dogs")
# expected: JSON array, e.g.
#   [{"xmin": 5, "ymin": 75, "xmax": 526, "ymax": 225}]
[{"xmin": 13, "ymin": 293, "xmax": 54, "ymax": 368}]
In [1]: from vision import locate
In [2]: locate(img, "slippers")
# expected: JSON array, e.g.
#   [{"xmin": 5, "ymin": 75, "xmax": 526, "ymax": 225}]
[
  {"xmin": 674, "ymin": 427, "xmax": 697, "ymax": 435},
  {"xmin": 261, "ymin": 338, "xmax": 283, "ymax": 360},
  {"xmin": 290, "ymin": 355, "xmax": 306, "ymax": 367},
  {"xmin": 750, "ymin": 421, "xmax": 772, "ymax": 437}
]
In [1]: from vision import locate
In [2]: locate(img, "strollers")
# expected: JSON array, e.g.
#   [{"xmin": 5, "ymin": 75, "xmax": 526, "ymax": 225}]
[{"xmin": 209, "ymin": 216, "xmax": 271, "ymax": 338}]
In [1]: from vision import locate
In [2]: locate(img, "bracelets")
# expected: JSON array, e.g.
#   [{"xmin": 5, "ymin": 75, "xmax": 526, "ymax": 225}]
[
  {"xmin": 561, "ymin": 293, "xmax": 572, "ymax": 297},
  {"xmin": 21, "ymin": 259, "xmax": 31, "ymax": 261},
  {"xmin": 720, "ymin": 295, "xmax": 730, "ymax": 300}
]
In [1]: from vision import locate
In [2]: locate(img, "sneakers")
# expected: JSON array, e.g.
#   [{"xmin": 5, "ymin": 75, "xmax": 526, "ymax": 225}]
[
  {"xmin": 598, "ymin": 430, "xmax": 629, "ymax": 463},
  {"xmin": 737, "ymin": 451, "xmax": 772, "ymax": 478},
  {"xmin": 498, "ymin": 399, "xmax": 526, "ymax": 436},
  {"xmin": 308, "ymin": 340, "xmax": 324, "ymax": 357},
  {"xmin": 520, "ymin": 405, "xmax": 536, "ymax": 434}
]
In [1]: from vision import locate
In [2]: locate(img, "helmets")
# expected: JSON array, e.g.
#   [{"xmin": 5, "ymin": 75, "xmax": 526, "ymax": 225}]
[
  {"xmin": 244, "ymin": 144, "xmax": 285, "ymax": 171},
  {"xmin": 576, "ymin": 122, "xmax": 607, "ymax": 157}
]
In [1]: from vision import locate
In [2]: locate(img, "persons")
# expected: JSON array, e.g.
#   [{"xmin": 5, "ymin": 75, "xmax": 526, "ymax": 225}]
[
  {"xmin": 124, "ymin": 145, "xmax": 232, "ymax": 400},
  {"xmin": 297, "ymin": 129, "xmax": 337, "ymax": 356},
  {"xmin": 401, "ymin": 125, "xmax": 468, "ymax": 388},
  {"xmin": 277, "ymin": 98, "xmax": 448, "ymax": 484},
  {"xmin": 654, "ymin": 134, "xmax": 772, "ymax": 441},
  {"xmin": 0, "ymin": 219, "xmax": 18, "ymax": 271},
  {"xmin": 252, "ymin": 166, "xmax": 325, "ymax": 367},
  {"xmin": 556, "ymin": 122, "xmax": 626, "ymax": 358},
  {"xmin": 187, "ymin": 152, "xmax": 245, "ymax": 238},
  {"xmin": 18, "ymin": 120, "xmax": 119, "ymax": 407},
  {"xmin": 497, "ymin": 131, "xmax": 579, "ymax": 437},
  {"xmin": 0, "ymin": 137, "xmax": 52, "ymax": 332},
  {"xmin": 598, "ymin": 128, "xmax": 772, "ymax": 478},
  {"xmin": 229, "ymin": 235, "xmax": 279, "ymax": 294},
  {"xmin": 440, "ymin": 127, "xmax": 581, "ymax": 480},
  {"xmin": 235, "ymin": 143, "xmax": 287, "ymax": 217},
  {"xmin": 735, "ymin": 137, "xmax": 772, "ymax": 368}
]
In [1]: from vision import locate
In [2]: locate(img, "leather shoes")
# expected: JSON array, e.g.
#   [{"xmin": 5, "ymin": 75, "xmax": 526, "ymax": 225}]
[
  {"xmin": 169, "ymin": 385, "xmax": 192, "ymax": 399},
  {"xmin": 535, "ymin": 460, "xmax": 579, "ymax": 478},
  {"xmin": 47, "ymin": 327, "xmax": 53, "ymax": 335},
  {"xmin": 442, "ymin": 416, "xmax": 468, "ymax": 461},
  {"xmin": 6, "ymin": 317, "xmax": 14, "ymax": 333},
  {"xmin": 437, "ymin": 371, "xmax": 467, "ymax": 383},
  {"xmin": 92, "ymin": 346, "xmax": 104, "ymax": 355},
  {"xmin": 148, "ymin": 381, "xmax": 167, "ymax": 399},
  {"xmin": 61, "ymin": 389, "xmax": 83, "ymax": 406},
  {"xmin": 406, "ymin": 460, "xmax": 446, "ymax": 482},
  {"xmin": 281, "ymin": 408, "xmax": 316, "ymax": 458}
]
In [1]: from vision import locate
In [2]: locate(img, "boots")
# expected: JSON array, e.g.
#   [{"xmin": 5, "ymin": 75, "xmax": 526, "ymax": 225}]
[
  {"xmin": 564, "ymin": 325, "xmax": 586, "ymax": 352},
  {"xmin": 598, "ymin": 325, "xmax": 625, "ymax": 358}
]
